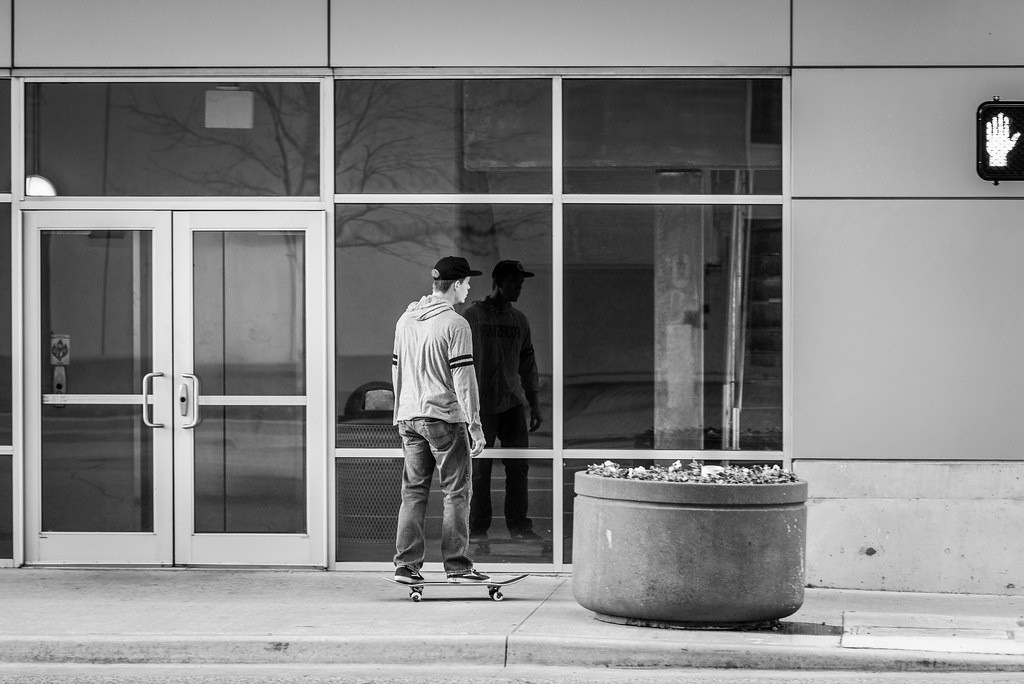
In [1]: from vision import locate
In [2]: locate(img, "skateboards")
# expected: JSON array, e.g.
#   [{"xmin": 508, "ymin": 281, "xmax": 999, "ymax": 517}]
[
  {"xmin": 467, "ymin": 535, "xmax": 572, "ymax": 557},
  {"xmin": 381, "ymin": 573, "xmax": 531, "ymax": 604}
]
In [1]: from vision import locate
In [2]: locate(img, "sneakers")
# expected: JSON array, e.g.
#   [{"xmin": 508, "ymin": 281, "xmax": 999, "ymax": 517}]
[
  {"xmin": 510, "ymin": 531, "xmax": 543, "ymax": 541},
  {"xmin": 468, "ymin": 533, "xmax": 488, "ymax": 542},
  {"xmin": 394, "ymin": 567, "xmax": 424, "ymax": 584},
  {"xmin": 446, "ymin": 569, "xmax": 491, "ymax": 583}
]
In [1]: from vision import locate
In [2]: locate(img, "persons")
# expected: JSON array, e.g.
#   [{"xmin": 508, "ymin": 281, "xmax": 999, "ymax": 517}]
[
  {"xmin": 462, "ymin": 261, "xmax": 544, "ymax": 541},
  {"xmin": 391, "ymin": 256, "xmax": 490, "ymax": 583}
]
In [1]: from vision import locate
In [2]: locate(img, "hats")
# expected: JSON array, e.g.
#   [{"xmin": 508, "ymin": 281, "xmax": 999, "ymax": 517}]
[
  {"xmin": 433, "ymin": 256, "xmax": 483, "ymax": 280},
  {"xmin": 491, "ymin": 260, "xmax": 535, "ymax": 280}
]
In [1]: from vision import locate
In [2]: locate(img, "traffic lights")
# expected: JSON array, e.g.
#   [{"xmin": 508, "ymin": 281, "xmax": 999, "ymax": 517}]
[{"xmin": 976, "ymin": 100, "xmax": 1024, "ymax": 181}]
